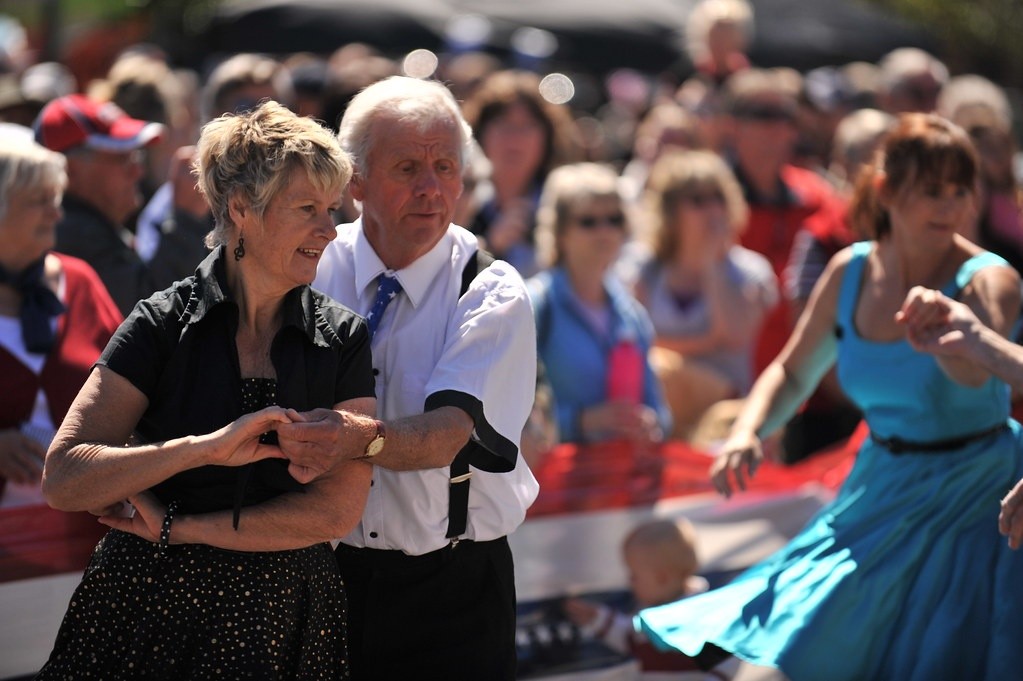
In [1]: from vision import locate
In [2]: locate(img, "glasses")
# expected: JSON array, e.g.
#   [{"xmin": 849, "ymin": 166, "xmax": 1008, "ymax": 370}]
[{"xmin": 565, "ymin": 211, "xmax": 627, "ymax": 229}]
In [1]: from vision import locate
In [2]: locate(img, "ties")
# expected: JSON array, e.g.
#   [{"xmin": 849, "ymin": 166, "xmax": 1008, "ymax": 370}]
[{"xmin": 365, "ymin": 274, "xmax": 403, "ymax": 344}]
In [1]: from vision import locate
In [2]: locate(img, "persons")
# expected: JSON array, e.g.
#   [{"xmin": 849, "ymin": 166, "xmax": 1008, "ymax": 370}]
[
  {"xmin": 564, "ymin": 514, "xmax": 729, "ymax": 681},
  {"xmin": 0, "ymin": 0, "xmax": 1023, "ymax": 486},
  {"xmin": 40, "ymin": 102, "xmax": 377, "ymax": 681},
  {"xmin": 313, "ymin": 78, "xmax": 540, "ymax": 681},
  {"xmin": 633, "ymin": 113, "xmax": 1023, "ymax": 680},
  {"xmin": 525, "ymin": 163, "xmax": 672, "ymax": 444}
]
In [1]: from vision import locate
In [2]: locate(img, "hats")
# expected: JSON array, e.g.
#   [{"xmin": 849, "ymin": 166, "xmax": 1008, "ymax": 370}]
[{"xmin": 32, "ymin": 91, "xmax": 164, "ymax": 153}]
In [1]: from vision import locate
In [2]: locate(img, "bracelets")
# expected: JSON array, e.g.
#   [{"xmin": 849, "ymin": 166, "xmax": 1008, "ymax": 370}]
[{"xmin": 158, "ymin": 502, "xmax": 177, "ymax": 564}]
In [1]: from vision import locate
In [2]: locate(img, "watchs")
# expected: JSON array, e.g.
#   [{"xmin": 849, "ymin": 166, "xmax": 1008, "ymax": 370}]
[{"xmin": 352, "ymin": 421, "xmax": 385, "ymax": 459}]
